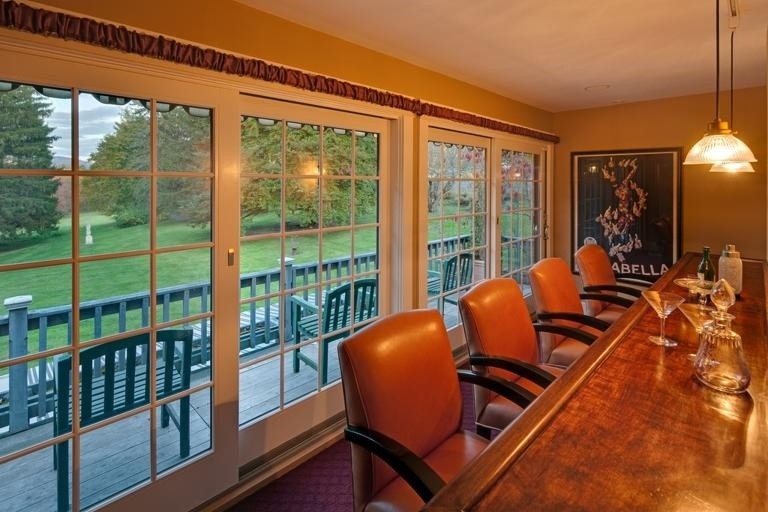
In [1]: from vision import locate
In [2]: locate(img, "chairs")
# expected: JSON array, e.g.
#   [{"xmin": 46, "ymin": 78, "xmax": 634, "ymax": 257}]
[
  {"xmin": 337, "ymin": 312, "xmax": 538, "ymax": 509},
  {"xmin": 526, "ymin": 255, "xmax": 618, "ymax": 377},
  {"xmin": 457, "ymin": 275, "xmax": 584, "ymax": 448},
  {"xmin": 575, "ymin": 243, "xmax": 637, "ymax": 335}
]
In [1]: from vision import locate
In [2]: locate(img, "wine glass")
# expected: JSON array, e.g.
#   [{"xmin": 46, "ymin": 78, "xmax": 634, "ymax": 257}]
[{"xmin": 641, "ymin": 278, "xmax": 736, "ymax": 366}]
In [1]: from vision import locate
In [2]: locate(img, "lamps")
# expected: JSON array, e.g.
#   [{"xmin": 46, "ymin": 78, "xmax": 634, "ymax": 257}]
[{"xmin": 681, "ymin": 0, "xmax": 758, "ymax": 176}]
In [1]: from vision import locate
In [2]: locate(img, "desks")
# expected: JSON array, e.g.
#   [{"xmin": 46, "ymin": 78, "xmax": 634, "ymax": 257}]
[{"xmin": 423, "ymin": 253, "xmax": 767, "ymax": 509}]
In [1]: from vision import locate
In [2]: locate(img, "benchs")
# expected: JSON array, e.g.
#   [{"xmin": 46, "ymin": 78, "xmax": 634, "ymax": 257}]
[
  {"xmin": 289, "ymin": 280, "xmax": 380, "ymax": 387},
  {"xmin": 54, "ymin": 328, "xmax": 196, "ymax": 510}
]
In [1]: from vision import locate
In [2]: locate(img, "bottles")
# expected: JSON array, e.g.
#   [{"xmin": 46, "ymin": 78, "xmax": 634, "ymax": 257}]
[{"xmin": 694, "ymin": 244, "xmax": 751, "ymax": 395}]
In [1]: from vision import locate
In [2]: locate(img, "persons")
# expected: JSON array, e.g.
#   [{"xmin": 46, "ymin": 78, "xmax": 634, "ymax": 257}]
[{"xmin": 290, "ymin": 238, "xmax": 299, "ymax": 256}]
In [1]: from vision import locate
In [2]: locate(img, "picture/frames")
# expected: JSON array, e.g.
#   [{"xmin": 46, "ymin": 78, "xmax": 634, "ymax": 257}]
[{"xmin": 568, "ymin": 148, "xmax": 686, "ymax": 287}]
